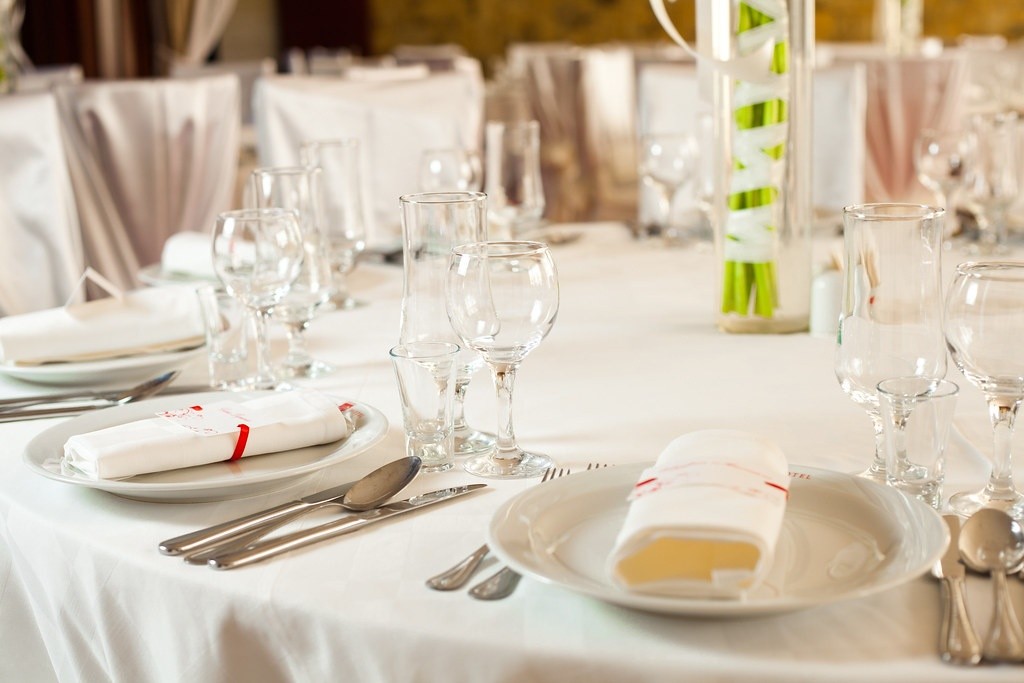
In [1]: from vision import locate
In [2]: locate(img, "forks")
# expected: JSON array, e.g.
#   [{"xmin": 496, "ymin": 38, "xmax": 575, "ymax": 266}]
[
  {"xmin": 469, "ymin": 463, "xmax": 615, "ymax": 600},
  {"xmin": 426, "ymin": 468, "xmax": 569, "ymax": 590}
]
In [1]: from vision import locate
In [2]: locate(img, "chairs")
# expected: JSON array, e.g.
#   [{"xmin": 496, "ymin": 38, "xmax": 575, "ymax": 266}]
[{"xmin": 0, "ymin": 35, "xmax": 1024, "ymax": 256}]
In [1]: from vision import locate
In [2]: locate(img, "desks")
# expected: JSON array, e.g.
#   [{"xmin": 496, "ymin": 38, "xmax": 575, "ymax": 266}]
[{"xmin": 0, "ymin": 230, "xmax": 1022, "ymax": 683}]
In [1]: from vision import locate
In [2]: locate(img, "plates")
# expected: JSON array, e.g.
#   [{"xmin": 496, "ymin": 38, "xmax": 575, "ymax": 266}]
[
  {"xmin": 136, "ymin": 262, "xmax": 225, "ymax": 289},
  {"xmin": 22, "ymin": 391, "xmax": 388, "ymax": 503},
  {"xmin": 0, "ymin": 311, "xmax": 232, "ymax": 385},
  {"xmin": 487, "ymin": 461, "xmax": 950, "ymax": 618}
]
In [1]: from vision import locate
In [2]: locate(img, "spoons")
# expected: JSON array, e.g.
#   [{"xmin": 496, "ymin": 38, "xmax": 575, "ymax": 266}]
[
  {"xmin": 959, "ymin": 508, "xmax": 1024, "ymax": 663},
  {"xmin": 183, "ymin": 456, "xmax": 421, "ymax": 564},
  {"xmin": 0, "ymin": 370, "xmax": 181, "ymax": 423}
]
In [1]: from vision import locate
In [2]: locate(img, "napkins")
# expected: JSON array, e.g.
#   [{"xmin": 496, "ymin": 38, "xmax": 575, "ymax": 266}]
[
  {"xmin": 607, "ymin": 427, "xmax": 790, "ymax": 596},
  {"xmin": 163, "ymin": 232, "xmax": 254, "ymax": 275},
  {"xmin": 60, "ymin": 391, "xmax": 349, "ymax": 479},
  {"xmin": 0, "ymin": 284, "xmax": 224, "ymax": 366}
]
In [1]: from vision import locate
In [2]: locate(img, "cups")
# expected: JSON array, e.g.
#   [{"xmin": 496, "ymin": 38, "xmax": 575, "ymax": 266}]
[
  {"xmin": 390, "ymin": 341, "xmax": 459, "ymax": 472},
  {"xmin": 631, "ymin": 54, "xmax": 717, "ymax": 245},
  {"xmin": 241, "ymin": 165, "xmax": 323, "ymax": 243},
  {"xmin": 878, "ymin": 377, "xmax": 959, "ymax": 499},
  {"xmin": 813, "ymin": 59, "xmax": 862, "ymax": 230},
  {"xmin": 199, "ymin": 284, "xmax": 257, "ymax": 391},
  {"xmin": 418, "ymin": 151, "xmax": 481, "ymax": 196}
]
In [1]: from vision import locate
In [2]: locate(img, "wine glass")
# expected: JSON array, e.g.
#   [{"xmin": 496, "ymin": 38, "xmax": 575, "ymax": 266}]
[
  {"xmin": 914, "ymin": 126, "xmax": 973, "ymax": 247},
  {"xmin": 299, "ymin": 138, "xmax": 367, "ymax": 312},
  {"xmin": 445, "ymin": 241, "xmax": 559, "ymax": 479},
  {"xmin": 485, "ymin": 121, "xmax": 545, "ymax": 240},
  {"xmin": 273, "ymin": 245, "xmax": 332, "ymax": 380},
  {"xmin": 944, "ymin": 263, "xmax": 1024, "ymax": 520},
  {"xmin": 835, "ymin": 203, "xmax": 945, "ymax": 489},
  {"xmin": 398, "ymin": 192, "xmax": 498, "ymax": 453},
  {"xmin": 214, "ymin": 209, "xmax": 303, "ymax": 387},
  {"xmin": 962, "ymin": 112, "xmax": 1021, "ymax": 261}
]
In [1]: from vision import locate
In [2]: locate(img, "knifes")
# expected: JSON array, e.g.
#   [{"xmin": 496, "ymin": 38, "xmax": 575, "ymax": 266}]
[
  {"xmin": 0, "ymin": 385, "xmax": 213, "ymax": 408},
  {"xmin": 931, "ymin": 514, "xmax": 981, "ymax": 664},
  {"xmin": 208, "ymin": 484, "xmax": 487, "ymax": 571},
  {"xmin": 158, "ymin": 480, "xmax": 359, "ymax": 554}
]
